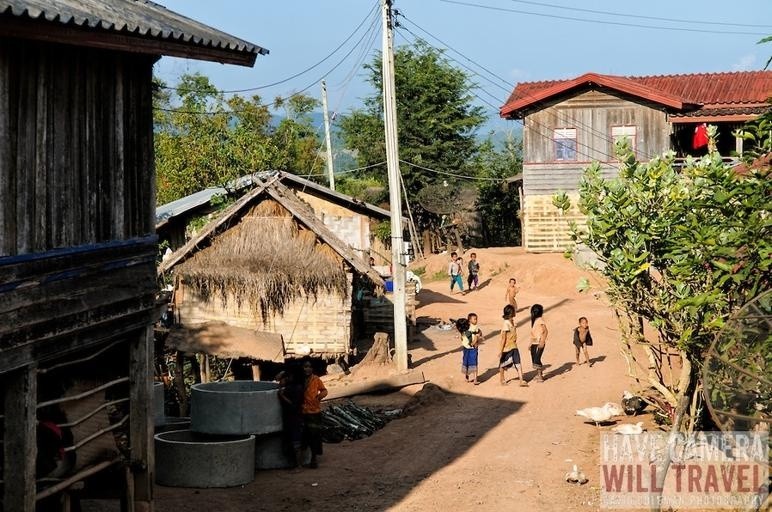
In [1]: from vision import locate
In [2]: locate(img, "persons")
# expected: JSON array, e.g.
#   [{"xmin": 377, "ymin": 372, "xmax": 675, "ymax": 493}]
[
  {"xmin": 370, "ymin": 256, "xmax": 375, "ymax": 265},
  {"xmin": 300, "ymin": 355, "xmax": 329, "ymax": 469},
  {"xmin": 528, "ymin": 303, "xmax": 550, "ymax": 384},
  {"xmin": 460, "ymin": 312, "xmax": 483, "ymax": 348},
  {"xmin": 455, "ymin": 318, "xmax": 485, "ymax": 386},
  {"xmin": 572, "ymin": 316, "xmax": 594, "ymax": 368},
  {"xmin": 273, "ymin": 368, "xmax": 309, "ymax": 475},
  {"xmin": 467, "ymin": 252, "xmax": 480, "ymax": 290},
  {"xmin": 504, "ymin": 277, "xmax": 521, "ymax": 311},
  {"xmin": 497, "ymin": 304, "xmax": 529, "ymax": 387},
  {"xmin": 448, "ymin": 251, "xmax": 467, "ymax": 295}
]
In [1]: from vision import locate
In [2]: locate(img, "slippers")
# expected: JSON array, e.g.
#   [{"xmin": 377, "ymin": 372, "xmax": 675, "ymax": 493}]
[{"xmin": 501, "ymin": 375, "xmax": 544, "ymax": 386}]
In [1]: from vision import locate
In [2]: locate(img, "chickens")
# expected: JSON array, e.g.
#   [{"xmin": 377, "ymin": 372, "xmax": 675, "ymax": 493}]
[
  {"xmin": 565, "ymin": 463, "xmax": 586, "ymax": 484},
  {"xmin": 577, "ymin": 390, "xmax": 649, "ymax": 435}
]
